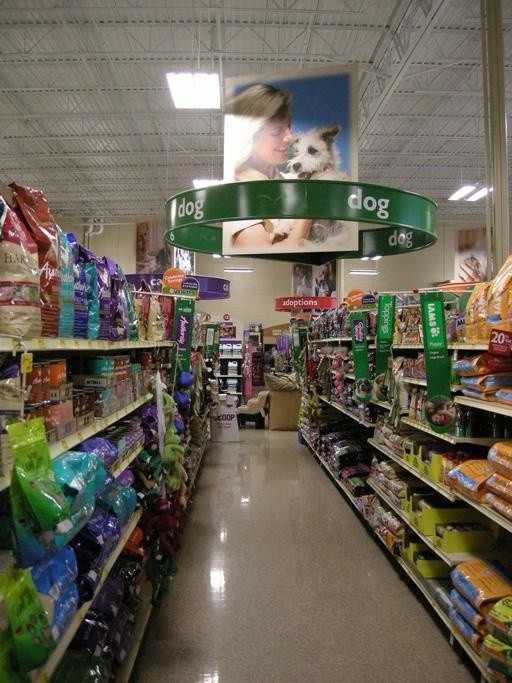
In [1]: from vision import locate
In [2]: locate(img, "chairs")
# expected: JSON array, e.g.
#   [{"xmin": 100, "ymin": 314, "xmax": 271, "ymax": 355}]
[{"xmin": 235, "ymin": 390, "xmax": 270, "ymax": 428}]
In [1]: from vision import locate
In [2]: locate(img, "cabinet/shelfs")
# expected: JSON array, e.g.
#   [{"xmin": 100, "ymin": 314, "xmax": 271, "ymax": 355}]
[{"xmin": 219, "ymin": 338, "xmax": 244, "ymax": 394}]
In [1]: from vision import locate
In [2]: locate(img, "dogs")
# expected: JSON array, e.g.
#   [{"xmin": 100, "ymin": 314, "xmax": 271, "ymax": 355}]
[
  {"xmin": 280, "ymin": 123, "xmax": 349, "ymax": 247},
  {"xmin": 374, "ymin": 374, "xmax": 387, "ymax": 395},
  {"xmin": 356, "ymin": 378, "xmax": 372, "ymax": 397}
]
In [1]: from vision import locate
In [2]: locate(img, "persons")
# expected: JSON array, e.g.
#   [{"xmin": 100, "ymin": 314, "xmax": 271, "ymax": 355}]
[
  {"xmin": 136, "ymin": 222, "xmax": 171, "ymax": 274},
  {"xmin": 192, "ymin": 345, "xmax": 209, "ymax": 390},
  {"xmin": 226, "ymin": 80, "xmax": 348, "ymax": 250},
  {"xmin": 263, "ymin": 345, "xmax": 273, "ymax": 377},
  {"xmin": 293, "ymin": 262, "xmax": 336, "ymax": 298},
  {"xmin": 425, "ymin": 398, "xmax": 456, "ymax": 426},
  {"xmin": 213, "ymin": 351, "xmax": 220, "ymax": 376}
]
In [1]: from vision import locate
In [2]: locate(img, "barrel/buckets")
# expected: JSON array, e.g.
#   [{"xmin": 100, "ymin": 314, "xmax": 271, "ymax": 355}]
[
  {"xmin": 218, "ymin": 344, "xmax": 223, "ymax": 354},
  {"xmin": 226, "ymin": 378, "xmax": 239, "ymax": 392},
  {"xmin": 219, "ymin": 394, "xmax": 226, "ymax": 404},
  {"xmin": 223, "ymin": 344, "xmax": 233, "ymax": 355},
  {"xmin": 232, "ymin": 344, "xmax": 243, "ymax": 356},
  {"xmin": 227, "ymin": 360, "xmax": 238, "ymax": 375},
  {"xmin": 210, "ymin": 379, "xmax": 219, "ymax": 389}
]
